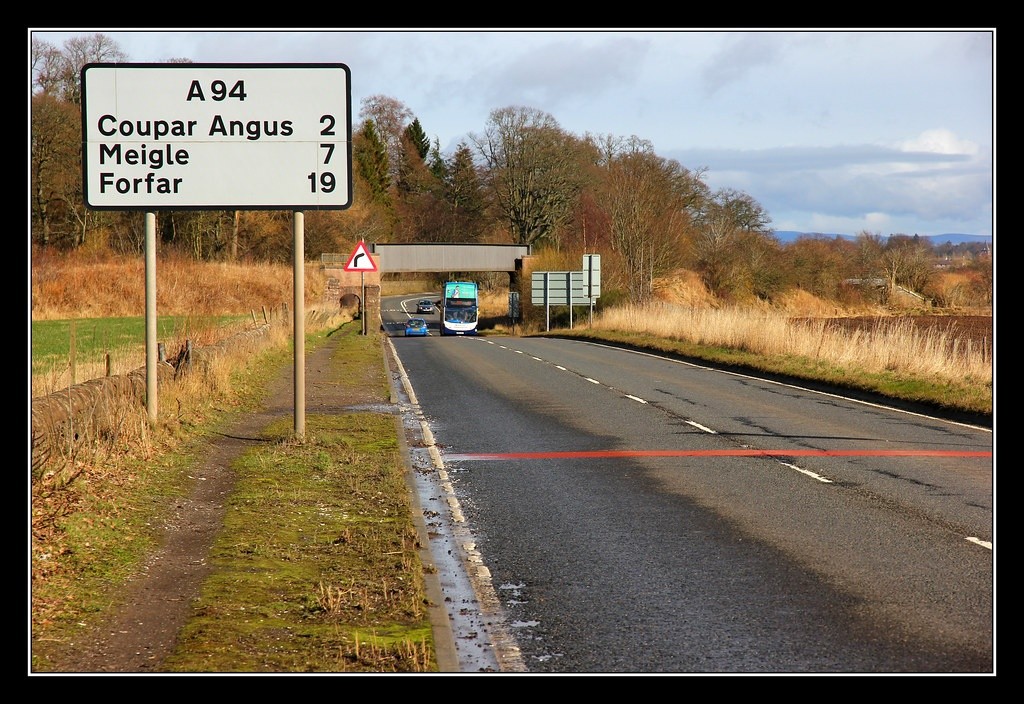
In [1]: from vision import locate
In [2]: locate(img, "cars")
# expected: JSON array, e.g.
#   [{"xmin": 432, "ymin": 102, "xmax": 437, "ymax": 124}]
[
  {"xmin": 403, "ymin": 317, "xmax": 430, "ymax": 337},
  {"xmin": 416, "ymin": 299, "xmax": 436, "ymax": 314}
]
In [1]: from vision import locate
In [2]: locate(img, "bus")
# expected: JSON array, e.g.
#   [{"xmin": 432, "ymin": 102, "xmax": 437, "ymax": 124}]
[{"xmin": 439, "ymin": 281, "xmax": 480, "ymax": 336}]
